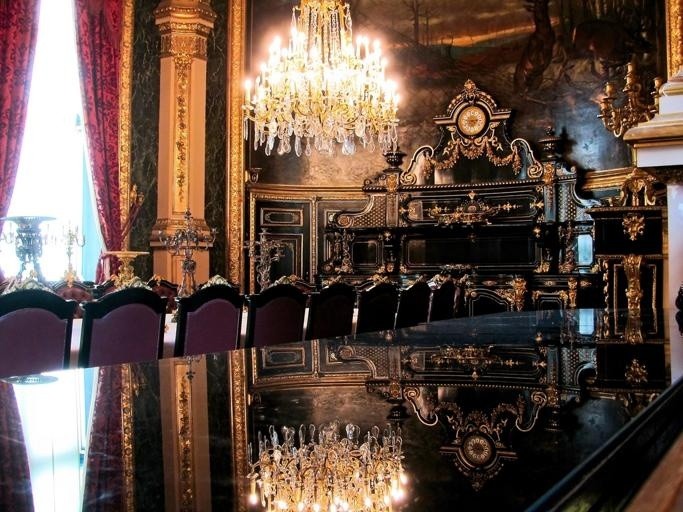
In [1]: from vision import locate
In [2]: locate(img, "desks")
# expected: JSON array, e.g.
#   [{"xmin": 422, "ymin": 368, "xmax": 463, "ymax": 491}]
[{"xmin": 0, "ymin": 309, "xmax": 683, "ymax": 512}]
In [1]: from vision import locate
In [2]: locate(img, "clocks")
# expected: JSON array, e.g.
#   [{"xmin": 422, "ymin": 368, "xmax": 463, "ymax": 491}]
[
  {"xmin": 457, "ymin": 106, "xmax": 486, "ymax": 136},
  {"xmin": 459, "ymin": 429, "xmax": 498, "ymax": 471}
]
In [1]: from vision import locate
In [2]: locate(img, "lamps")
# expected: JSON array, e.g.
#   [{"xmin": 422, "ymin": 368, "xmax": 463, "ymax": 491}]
[
  {"xmin": 245, "ymin": 421, "xmax": 407, "ymax": 512},
  {"xmin": 241, "ymin": 0, "xmax": 400, "ymax": 157}
]
